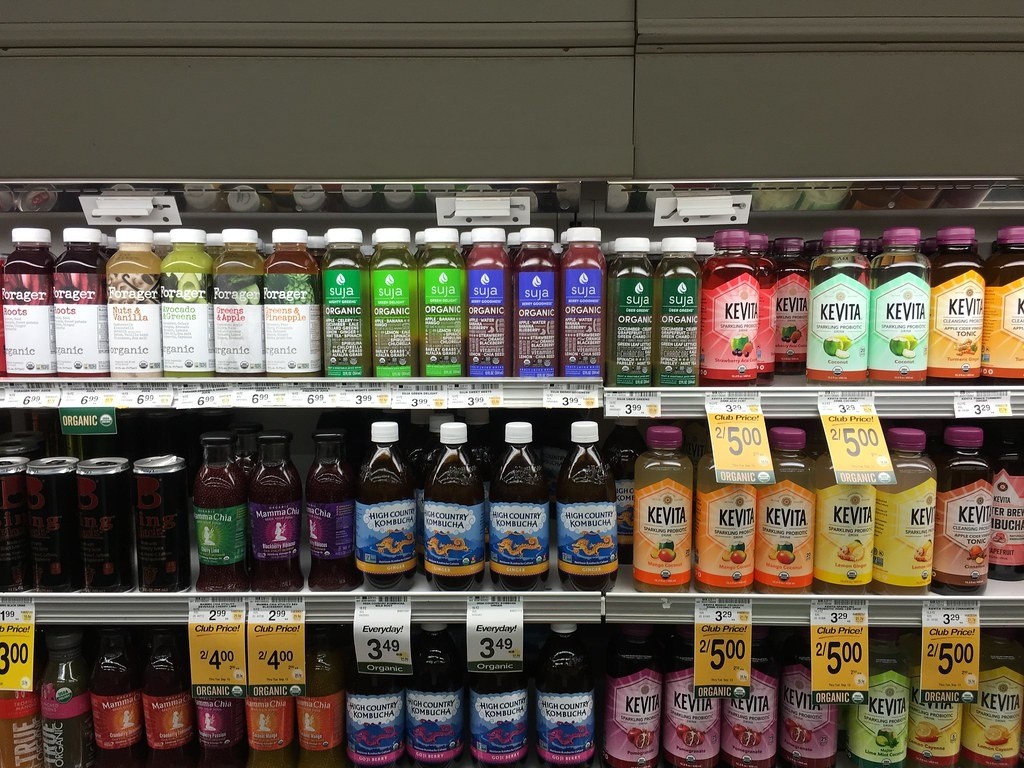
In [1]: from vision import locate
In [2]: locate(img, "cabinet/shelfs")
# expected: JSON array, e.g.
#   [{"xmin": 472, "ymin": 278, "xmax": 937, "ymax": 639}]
[{"xmin": 0, "ymin": 169, "xmax": 1024, "ymax": 626}]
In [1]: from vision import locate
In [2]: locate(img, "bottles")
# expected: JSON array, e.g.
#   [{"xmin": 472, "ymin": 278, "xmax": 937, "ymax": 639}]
[
  {"xmin": 355, "ymin": 407, "xmax": 649, "ymax": 590},
  {"xmin": 700, "ymin": 227, "xmax": 1024, "ymax": 385},
  {"xmin": 601, "ymin": 236, "xmax": 716, "ymax": 387},
  {"xmin": 265, "ymin": 227, "xmax": 605, "ymax": 377},
  {"xmin": 0, "ymin": 622, "xmax": 596, "ymax": 768},
  {"xmin": 3, "ymin": 229, "xmax": 56, "ymax": 377},
  {"xmin": 601, "ymin": 623, "xmax": 1024, "ymax": 768},
  {"xmin": 614, "ymin": 425, "xmax": 1024, "ymax": 595},
  {"xmin": 192, "ymin": 423, "xmax": 365, "ymax": 592},
  {"xmin": 54, "ymin": 228, "xmax": 266, "ymax": 377}
]
